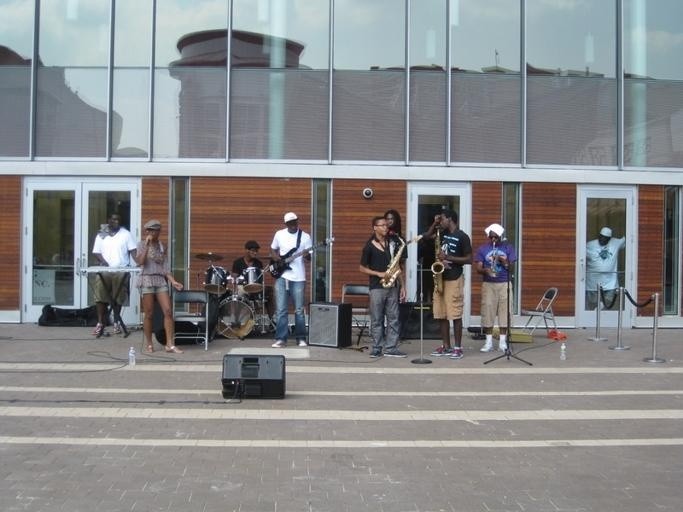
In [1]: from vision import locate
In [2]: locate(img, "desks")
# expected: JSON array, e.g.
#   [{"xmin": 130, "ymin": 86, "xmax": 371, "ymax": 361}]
[{"xmin": 81, "ymin": 266, "xmax": 143, "ymax": 337}]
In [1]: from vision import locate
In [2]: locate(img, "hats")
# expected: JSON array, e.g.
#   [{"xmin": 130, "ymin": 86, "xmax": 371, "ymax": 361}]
[
  {"xmin": 599, "ymin": 226, "xmax": 612, "ymax": 238},
  {"xmin": 144, "ymin": 219, "xmax": 162, "ymax": 231},
  {"xmin": 284, "ymin": 212, "xmax": 299, "ymax": 224}
]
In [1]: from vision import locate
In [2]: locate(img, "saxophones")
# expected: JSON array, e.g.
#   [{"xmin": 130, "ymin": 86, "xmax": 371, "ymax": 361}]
[
  {"xmin": 430, "ymin": 226, "xmax": 445, "ymax": 295},
  {"xmin": 379, "ymin": 231, "xmax": 405, "ymax": 288}
]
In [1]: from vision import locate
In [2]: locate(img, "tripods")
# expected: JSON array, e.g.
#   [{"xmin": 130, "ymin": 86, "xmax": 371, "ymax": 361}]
[
  {"xmin": 483, "ymin": 257, "xmax": 533, "ymax": 366},
  {"xmin": 255, "ymin": 264, "xmax": 276, "ymax": 333}
]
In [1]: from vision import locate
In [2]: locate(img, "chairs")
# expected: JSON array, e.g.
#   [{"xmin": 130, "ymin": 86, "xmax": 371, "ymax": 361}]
[
  {"xmin": 173, "ymin": 290, "xmax": 210, "ymax": 350},
  {"xmin": 249, "ymin": 286, "xmax": 276, "ymax": 329},
  {"xmin": 522, "ymin": 286, "xmax": 559, "ymax": 335},
  {"xmin": 341, "ymin": 284, "xmax": 372, "ymax": 346}
]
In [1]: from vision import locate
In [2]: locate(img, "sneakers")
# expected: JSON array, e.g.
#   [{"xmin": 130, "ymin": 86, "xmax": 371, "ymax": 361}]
[
  {"xmin": 92, "ymin": 322, "xmax": 105, "ymax": 336},
  {"xmin": 142, "ymin": 344, "xmax": 154, "ymax": 353},
  {"xmin": 480, "ymin": 342, "xmax": 495, "ymax": 352},
  {"xmin": 450, "ymin": 347, "xmax": 464, "ymax": 360},
  {"xmin": 164, "ymin": 345, "xmax": 185, "ymax": 353},
  {"xmin": 498, "ymin": 341, "xmax": 508, "ymax": 352},
  {"xmin": 112, "ymin": 321, "xmax": 122, "ymax": 335},
  {"xmin": 296, "ymin": 339, "xmax": 308, "ymax": 348},
  {"xmin": 383, "ymin": 349, "xmax": 408, "ymax": 358},
  {"xmin": 272, "ymin": 338, "xmax": 288, "ymax": 348},
  {"xmin": 430, "ymin": 344, "xmax": 453, "ymax": 356},
  {"xmin": 368, "ymin": 347, "xmax": 384, "ymax": 358}
]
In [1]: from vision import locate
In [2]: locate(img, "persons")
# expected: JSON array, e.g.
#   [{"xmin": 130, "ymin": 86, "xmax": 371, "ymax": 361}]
[
  {"xmin": 425, "ymin": 207, "xmax": 474, "ymax": 359},
  {"xmin": 474, "ymin": 223, "xmax": 517, "ymax": 352},
  {"xmin": 270, "ymin": 212, "xmax": 314, "ymax": 348},
  {"xmin": 91, "ymin": 210, "xmax": 137, "ymax": 335},
  {"xmin": 232, "ymin": 240, "xmax": 276, "ymax": 332},
  {"xmin": 586, "ymin": 227, "xmax": 626, "ymax": 311},
  {"xmin": 136, "ymin": 219, "xmax": 184, "ymax": 354},
  {"xmin": 360, "ymin": 209, "xmax": 408, "ymax": 357}
]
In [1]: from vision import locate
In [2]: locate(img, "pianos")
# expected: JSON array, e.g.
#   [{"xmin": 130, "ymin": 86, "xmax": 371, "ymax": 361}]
[{"xmin": 80, "ymin": 265, "xmax": 141, "ymax": 272}]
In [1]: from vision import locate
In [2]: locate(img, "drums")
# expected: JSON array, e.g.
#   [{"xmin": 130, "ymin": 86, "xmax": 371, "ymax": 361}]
[
  {"xmin": 203, "ymin": 266, "xmax": 230, "ymax": 295},
  {"xmin": 245, "ymin": 285, "xmax": 273, "ymax": 305},
  {"xmin": 215, "ymin": 294, "xmax": 256, "ymax": 339},
  {"xmin": 242, "ymin": 266, "xmax": 263, "ymax": 293}
]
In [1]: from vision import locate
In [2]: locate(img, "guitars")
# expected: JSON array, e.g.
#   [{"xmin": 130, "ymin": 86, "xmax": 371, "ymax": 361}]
[{"xmin": 269, "ymin": 237, "xmax": 334, "ymax": 279}]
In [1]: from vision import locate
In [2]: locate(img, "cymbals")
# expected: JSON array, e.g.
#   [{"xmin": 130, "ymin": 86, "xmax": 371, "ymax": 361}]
[{"xmin": 195, "ymin": 254, "xmax": 224, "ymax": 260}]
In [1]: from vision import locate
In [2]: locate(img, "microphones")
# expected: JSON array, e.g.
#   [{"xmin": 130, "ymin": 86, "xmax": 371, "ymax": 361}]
[{"xmin": 147, "ymin": 239, "xmax": 150, "ymax": 245}]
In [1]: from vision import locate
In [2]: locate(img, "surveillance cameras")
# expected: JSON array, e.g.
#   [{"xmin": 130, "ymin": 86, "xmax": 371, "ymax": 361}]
[{"xmin": 362, "ymin": 188, "xmax": 373, "ymax": 198}]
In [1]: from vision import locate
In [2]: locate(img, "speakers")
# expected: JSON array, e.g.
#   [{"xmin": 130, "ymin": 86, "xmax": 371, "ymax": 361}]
[
  {"xmin": 221, "ymin": 354, "xmax": 285, "ymax": 400},
  {"xmin": 398, "ymin": 301, "xmax": 444, "ymax": 339},
  {"xmin": 308, "ymin": 301, "xmax": 352, "ymax": 348},
  {"xmin": 155, "ymin": 321, "xmax": 197, "ymax": 346}
]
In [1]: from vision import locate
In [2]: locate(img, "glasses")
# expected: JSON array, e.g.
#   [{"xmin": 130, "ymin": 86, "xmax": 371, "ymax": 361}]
[
  {"xmin": 490, "ymin": 236, "xmax": 498, "ymax": 240},
  {"xmin": 375, "ymin": 224, "xmax": 389, "ymax": 227}
]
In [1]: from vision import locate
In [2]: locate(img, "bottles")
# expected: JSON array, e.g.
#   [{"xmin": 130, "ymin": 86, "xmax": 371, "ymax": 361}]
[
  {"xmin": 560, "ymin": 342, "xmax": 567, "ymax": 360},
  {"xmin": 127, "ymin": 345, "xmax": 135, "ymax": 367}
]
